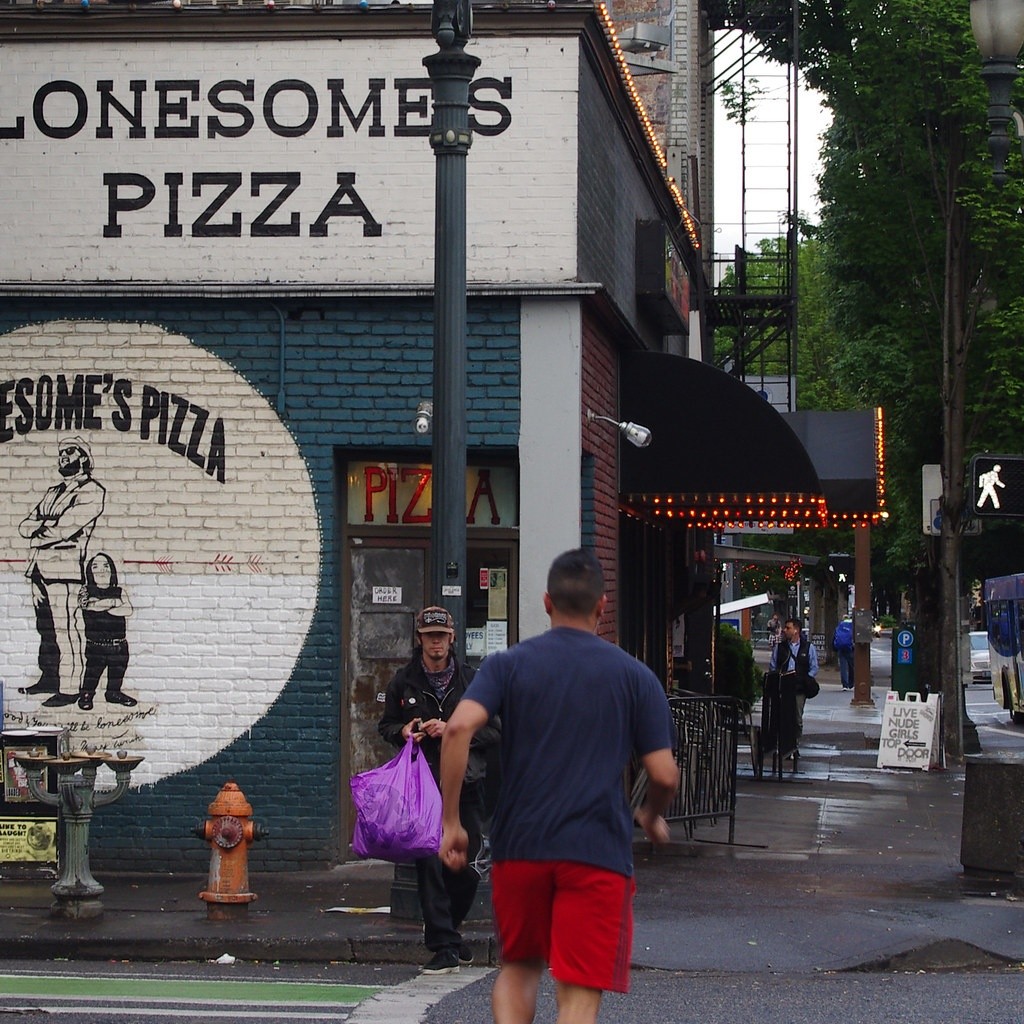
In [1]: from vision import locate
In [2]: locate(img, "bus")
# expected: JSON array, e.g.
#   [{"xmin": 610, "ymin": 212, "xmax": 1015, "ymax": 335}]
[{"xmin": 982, "ymin": 573, "xmax": 1024, "ymax": 724}]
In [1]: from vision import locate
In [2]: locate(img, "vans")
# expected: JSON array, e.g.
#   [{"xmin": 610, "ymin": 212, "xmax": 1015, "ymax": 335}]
[{"xmin": 968, "ymin": 631, "xmax": 992, "ymax": 684}]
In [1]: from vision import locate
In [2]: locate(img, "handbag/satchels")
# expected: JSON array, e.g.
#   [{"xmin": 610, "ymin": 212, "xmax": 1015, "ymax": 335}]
[
  {"xmin": 797, "ymin": 672, "xmax": 820, "ymax": 699},
  {"xmin": 349, "ymin": 733, "xmax": 443, "ymax": 864}
]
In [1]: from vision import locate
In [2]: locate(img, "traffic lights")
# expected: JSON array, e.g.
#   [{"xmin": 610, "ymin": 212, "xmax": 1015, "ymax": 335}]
[{"xmin": 967, "ymin": 453, "xmax": 1024, "ymax": 516}]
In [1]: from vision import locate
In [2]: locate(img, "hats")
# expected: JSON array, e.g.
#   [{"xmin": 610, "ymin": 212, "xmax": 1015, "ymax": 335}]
[{"xmin": 415, "ymin": 606, "xmax": 454, "ymax": 634}]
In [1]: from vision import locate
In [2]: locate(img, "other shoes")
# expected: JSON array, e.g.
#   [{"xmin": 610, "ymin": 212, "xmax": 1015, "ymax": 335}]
[
  {"xmin": 423, "ymin": 950, "xmax": 460, "ymax": 974},
  {"xmin": 792, "ymin": 750, "xmax": 801, "ymax": 759},
  {"xmin": 458, "ymin": 944, "xmax": 473, "ymax": 964},
  {"xmin": 849, "ymin": 686, "xmax": 853, "ymax": 690},
  {"xmin": 842, "ymin": 686, "xmax": 848, "ymax": 691}
]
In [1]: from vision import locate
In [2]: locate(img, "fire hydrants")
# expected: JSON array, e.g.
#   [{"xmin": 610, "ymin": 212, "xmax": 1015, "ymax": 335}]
[{"xmin": 190, "ymin": 781, "xmax": 268, "ymax": 921}]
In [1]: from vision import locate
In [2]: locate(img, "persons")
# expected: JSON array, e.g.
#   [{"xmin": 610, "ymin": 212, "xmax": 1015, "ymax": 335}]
[
  {"xmin": 768, "ymin": 619, "xmax": 819, "ymax": 759},
  {"xmin": 768, "ymin": 612, "xmax": 782, "ymax": 647},
  {"xmin": 831, "ymin": 615, "xmax": 855, "ymax": 691},
  {"xmin": 438, "ymin": 545, "xmax": 680, "ymax": 1024},
  {"xmin": 380, "ymin": 608, "xmax": 503, "ymax": 977}
]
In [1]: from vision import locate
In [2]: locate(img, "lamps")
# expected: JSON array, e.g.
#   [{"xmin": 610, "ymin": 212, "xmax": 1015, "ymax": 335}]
[
  {"xmin": 588, "ymin": 410, "xmax": 652, "ymax": 448},
  {"xmin": 411, "ymin": 401, "xmax": 433, "ymax": 438}
]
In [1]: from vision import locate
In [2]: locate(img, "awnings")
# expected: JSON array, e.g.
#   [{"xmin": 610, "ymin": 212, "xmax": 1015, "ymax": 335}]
[
  {"xmin": 714, "ymin": 543, "xmax": 822, "ymax": 567},
  {"xmin": 618, "ymin": 346, "xmax": 828, "ymax": 529},
  {"xmin": 781, "ymin": 408, "xmax": 887, "ymax": 529}
]
